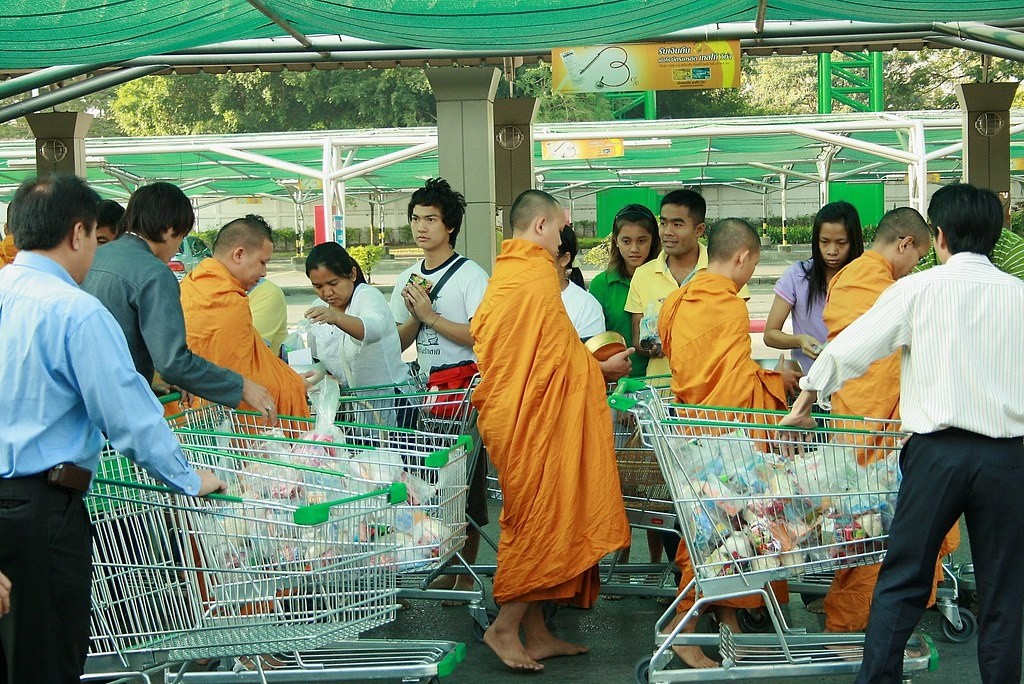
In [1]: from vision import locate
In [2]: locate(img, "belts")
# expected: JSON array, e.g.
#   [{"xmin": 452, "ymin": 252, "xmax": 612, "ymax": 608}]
[{"xmin": 931, "ymin": 428, "xmax": 989, "ymax": 438}]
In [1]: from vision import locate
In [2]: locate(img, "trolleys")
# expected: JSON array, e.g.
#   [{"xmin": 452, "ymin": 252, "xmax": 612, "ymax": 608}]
[
  {"xmin": 598, "ymin": 374, "xmax": 979, "ymax": 684},
  {"xmin": 80, "ymin": 377, "xmax": 687, "ymax": 684}
]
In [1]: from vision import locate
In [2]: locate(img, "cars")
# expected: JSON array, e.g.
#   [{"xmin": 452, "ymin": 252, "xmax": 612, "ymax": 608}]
[{"xmin": 168, "ymin": 235, "xmax": 213, "ymax": 283}]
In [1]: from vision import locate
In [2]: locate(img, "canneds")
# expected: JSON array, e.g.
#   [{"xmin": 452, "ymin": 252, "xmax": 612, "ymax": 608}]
[{"xmin": 639, "ymin": 313, "xmax": 658, "ymax": 350}]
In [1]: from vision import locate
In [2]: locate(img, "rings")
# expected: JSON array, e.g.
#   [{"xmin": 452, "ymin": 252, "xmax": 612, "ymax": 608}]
[{"xmin": 265, "ymin": 405, "xmax": 273, "ymax": 412}]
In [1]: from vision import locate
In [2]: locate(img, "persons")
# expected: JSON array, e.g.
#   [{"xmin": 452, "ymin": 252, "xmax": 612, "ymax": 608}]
[
  {"xmin": 663, "ymin": 219, "xmax": 801, "ymax": 666},
  {"xmin": 778, "ymin": 183, "xmax": 1024, "ymax": 684},
  {"xmin": 0, "ymin": 175, "xmax": 227, "ymax": 684},
  {"xmin": 823, "ymin": 208, "xmax": 962, "ymax": 662},
  {"xmin": 1, "ymin": 181, "xmax": 1024, "ymax": 604},
  {"xmin": 467, "ymin": 192, "xmax": 633, "ymax": 673}
]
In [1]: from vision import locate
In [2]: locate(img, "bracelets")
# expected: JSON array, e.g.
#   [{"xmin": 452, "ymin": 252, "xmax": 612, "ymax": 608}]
[{"xmin": 429, "ymin": 315, "xmax": 441, "ymax": 328}]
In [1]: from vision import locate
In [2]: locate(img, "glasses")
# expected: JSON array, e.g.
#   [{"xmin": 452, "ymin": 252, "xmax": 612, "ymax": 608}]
[{"xmin": 900, "ymin": 236, "xmax": 926, "ymax": 265}]
